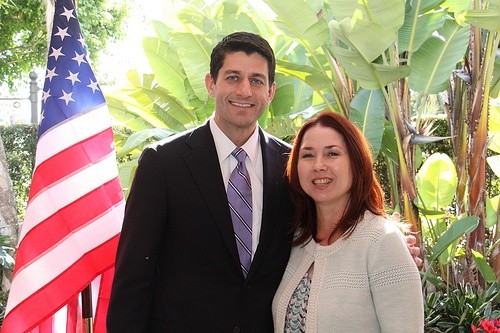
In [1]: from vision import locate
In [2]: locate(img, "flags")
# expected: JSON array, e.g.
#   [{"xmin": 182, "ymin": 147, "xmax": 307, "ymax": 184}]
[{"xmin": 0, "ymin": 0, "xmax": 126, "ymax": 333}]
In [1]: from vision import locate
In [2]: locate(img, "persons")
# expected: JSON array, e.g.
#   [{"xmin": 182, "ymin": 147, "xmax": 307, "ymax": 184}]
[
  {"xmin": 105, "ymin": 32, "xmax": 425, "ymax": 333},
  {"xmin": 271, "ymin": 111, "xmax": 428, "ymax": 333}
]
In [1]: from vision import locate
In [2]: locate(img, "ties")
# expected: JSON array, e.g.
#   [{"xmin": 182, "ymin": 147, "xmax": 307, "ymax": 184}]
[{"xmin": 227, "ymin": 149, "xmax": 252, "ymax": 278}]
